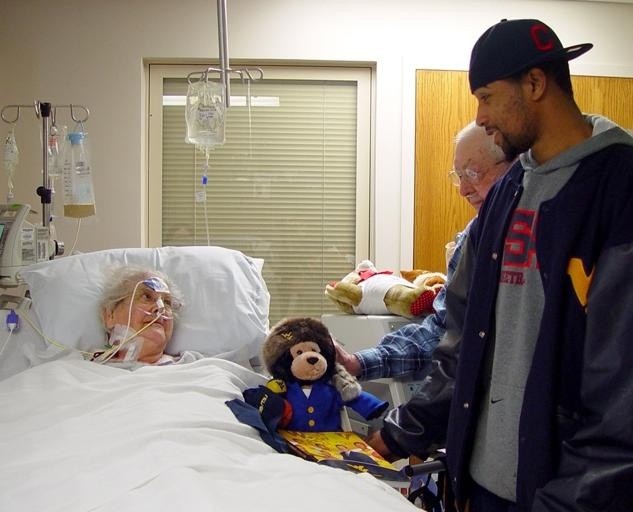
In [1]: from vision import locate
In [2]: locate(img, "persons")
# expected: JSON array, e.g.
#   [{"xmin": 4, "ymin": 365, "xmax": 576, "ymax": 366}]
[
  {"xmin": 80, "ymin": 263, "xmax": 207, "ymax": 375},
  {"xmin": 354, "ymin": 14, "xmax": 632, "ymax": 512},
  {"xmin": 313, "ymin": 118, "xmax": 508, "ymax": 384}
]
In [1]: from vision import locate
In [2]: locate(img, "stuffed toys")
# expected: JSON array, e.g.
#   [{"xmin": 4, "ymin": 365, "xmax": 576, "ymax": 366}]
[{"xmin": 245, "ymin": 315, "xmax": 387, "ymax": 430}]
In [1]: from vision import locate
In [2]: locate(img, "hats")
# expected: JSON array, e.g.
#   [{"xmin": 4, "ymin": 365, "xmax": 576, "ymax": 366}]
[{"xmin": 469, "ymin": 19, "xmax": 593, "ymax": 94}]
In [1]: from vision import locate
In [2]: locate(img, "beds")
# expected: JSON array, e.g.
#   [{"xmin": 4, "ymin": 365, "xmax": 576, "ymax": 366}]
[{"xmin": 1, "ymin": 306, "xmax": 420, "ymax": 511}]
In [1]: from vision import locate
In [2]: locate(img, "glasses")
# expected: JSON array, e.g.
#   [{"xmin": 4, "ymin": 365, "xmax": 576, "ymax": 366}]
[{"xmin": 447, "ymin": 161, "xmax": 503, "ymax": 186}]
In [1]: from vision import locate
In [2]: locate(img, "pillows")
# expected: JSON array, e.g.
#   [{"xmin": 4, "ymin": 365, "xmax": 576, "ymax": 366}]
[{"xmin": 22, "ymin": 246, "xmax": 272, "ymax": 363}]
